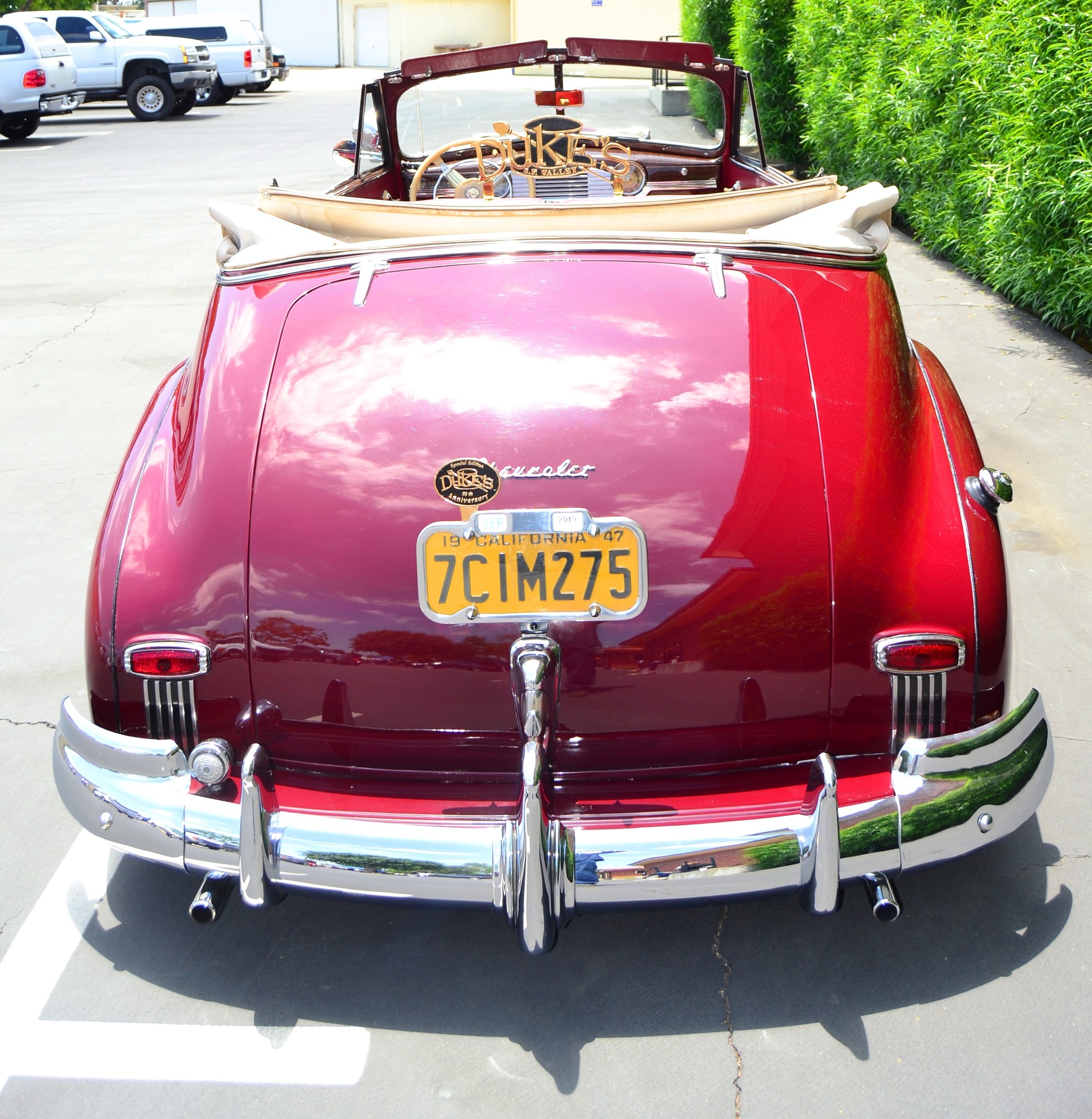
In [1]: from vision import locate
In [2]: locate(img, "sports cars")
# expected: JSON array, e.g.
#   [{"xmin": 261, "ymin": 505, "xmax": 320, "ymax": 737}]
[{"xmin": 53, "ymin": 38, "xmax": 1056, "ymax": 959}]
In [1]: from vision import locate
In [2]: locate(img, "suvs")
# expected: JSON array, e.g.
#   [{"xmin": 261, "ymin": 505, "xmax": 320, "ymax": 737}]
[
  {"xmin": 6, "ymin": 10, "xmax": 219, "ymax": 123},
  {"xmin": 124, "ymin": 17, "xmax": 275, "ymax": 106},
  {"xmin": 0, "ymin": 14, "xmax": 89, "ymax": 139}
]
